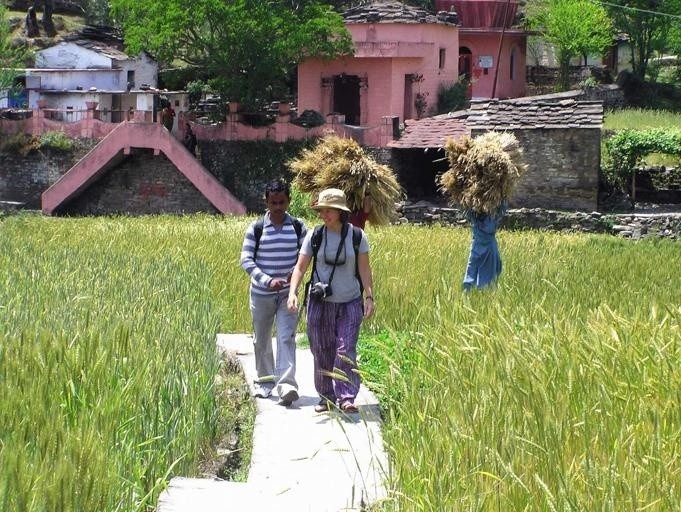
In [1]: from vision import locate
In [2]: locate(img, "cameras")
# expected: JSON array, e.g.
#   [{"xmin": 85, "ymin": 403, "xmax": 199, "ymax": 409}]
[{"xmin": 310, "ymin": 282, "xmax": 333, "ymax": 302}]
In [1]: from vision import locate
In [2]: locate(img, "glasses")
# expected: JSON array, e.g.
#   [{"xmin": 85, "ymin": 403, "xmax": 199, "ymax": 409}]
[{"xmin": 323, "ymin": 252, "xmax": 346, "ymax": 265}]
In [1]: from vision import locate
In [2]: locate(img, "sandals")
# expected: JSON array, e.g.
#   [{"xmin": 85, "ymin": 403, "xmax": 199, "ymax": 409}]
[{"xmin": 315, "ymin": 402, "xmax": 359, "ymax": 413}]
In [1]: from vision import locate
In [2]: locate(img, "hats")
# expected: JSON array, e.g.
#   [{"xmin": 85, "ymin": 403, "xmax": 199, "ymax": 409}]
[{"xmin": 312, "ymin": 188, "xmax": 352, "ymax": 214}]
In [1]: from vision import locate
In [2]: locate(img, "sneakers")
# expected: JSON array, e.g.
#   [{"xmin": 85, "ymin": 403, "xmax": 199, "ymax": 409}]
[
  {"xmin": 278, "ymin": 387, "xmax": 299, "ymax": 406},
  {"xmin": 253, "ymin": 383, "xmax": 273, "ymax": 398}
]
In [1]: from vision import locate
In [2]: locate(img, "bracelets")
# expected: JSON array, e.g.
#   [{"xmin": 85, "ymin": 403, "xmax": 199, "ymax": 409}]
[{"xmin": 365, "ymin": 294, "xmax": 375, "ymax": 301}]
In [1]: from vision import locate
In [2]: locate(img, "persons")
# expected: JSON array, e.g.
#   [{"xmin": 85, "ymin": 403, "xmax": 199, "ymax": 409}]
[
  {"xmin": 344, "ymin": 187, "xmax": 373, "ymax": 232},
  {"xmin": 161, "ymin": 102, "xmax": 177, "ymax": 133},
  {"xmin": 464, "ymin": 195, "xmax": 509, "ymax": 295},
  {"xmin": 288, "ymin": 188, "xmax": 377, "ymax": 415},
  {"xmin": 238, "ymin": 180, "xmax": 309, "ymax": 407}
]
